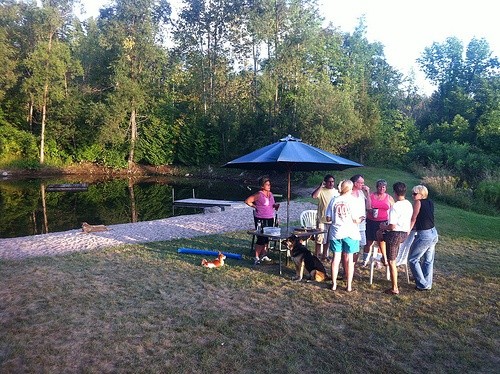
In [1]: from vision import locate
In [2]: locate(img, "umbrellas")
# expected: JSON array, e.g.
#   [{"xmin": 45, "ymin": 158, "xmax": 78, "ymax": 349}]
[{"xmin": 220, "ymin": 134, "xmax": 364, "ymax": 267}]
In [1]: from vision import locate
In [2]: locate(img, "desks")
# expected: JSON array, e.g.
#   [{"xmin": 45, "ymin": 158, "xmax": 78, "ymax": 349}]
[{"xmin": 247, "ymin": 225, "xmax": 328, "ymax": 274}]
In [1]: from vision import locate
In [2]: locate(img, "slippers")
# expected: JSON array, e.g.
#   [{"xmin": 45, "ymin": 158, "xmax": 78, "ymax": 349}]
[
  {"xmin": 385, "ymin": 288, "xmax": 399, "ymax": 295},
  {"xmin": 381, "ymin": 257, "xmax": 388, "ymax": 266}
]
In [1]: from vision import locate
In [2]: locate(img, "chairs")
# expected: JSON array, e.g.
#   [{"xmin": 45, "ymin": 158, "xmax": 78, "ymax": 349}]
[
  {"xmin": 300, "ymin": 210, "xmax": 319, "ymax": 249},
  {"xmin": 370, "ymin": 229, "xmax": 417, "ymax": 284}
]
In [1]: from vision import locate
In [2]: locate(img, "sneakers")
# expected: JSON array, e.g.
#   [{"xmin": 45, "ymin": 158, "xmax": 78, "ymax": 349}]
[
  {"xmin": 255, "ymin": 258, "xmax": 260, "ymax": 264},
  {"xmin": 261, "ymin": 256, "xmax": 272, "ymax": 262}
]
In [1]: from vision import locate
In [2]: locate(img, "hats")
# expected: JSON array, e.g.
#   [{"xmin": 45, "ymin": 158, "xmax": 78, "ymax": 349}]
[{"xmin": 259, "ymin": 177, "xmax": 270, "ymax": 186}]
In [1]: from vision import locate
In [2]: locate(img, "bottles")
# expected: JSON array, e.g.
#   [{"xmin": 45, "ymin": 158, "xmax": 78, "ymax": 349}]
[
  {"xmin": 257, "ymin": 220, "xmax": 261, "ymax": 233},
  {"xmin": 275, "ymin": 214, "xmax": 278, "ymax": 227}
]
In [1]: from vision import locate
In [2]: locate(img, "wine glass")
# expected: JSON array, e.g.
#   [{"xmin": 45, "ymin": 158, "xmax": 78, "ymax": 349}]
[{"xmin": 381, "ymin": 222, "xmax": 386, "ymax": 234}]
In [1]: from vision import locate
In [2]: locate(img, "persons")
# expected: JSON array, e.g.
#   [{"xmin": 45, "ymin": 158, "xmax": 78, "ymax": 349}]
[
  {"xmin": 245, "ymin": 176, "xmax": 275, "ymax": 265},
  {"xmin": 408, "ymin": 185, "xmax": 439, "ymax": 291},
  {"xmin": 376, "ymin": 182, "xmax": 414, "ymax": 295},
  {"xmin": 326, "ymin": 179, "xmax": 365, "ymax": 291},
  {"xmin": 312, "ymin": 174, "xmax": 395, "ymax": 267}
]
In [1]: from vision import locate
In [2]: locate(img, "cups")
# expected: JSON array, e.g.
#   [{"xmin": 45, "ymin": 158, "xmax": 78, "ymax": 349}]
[{"xmin": 373, "ymin": 208, "xmax": 378, "ymax": 218}]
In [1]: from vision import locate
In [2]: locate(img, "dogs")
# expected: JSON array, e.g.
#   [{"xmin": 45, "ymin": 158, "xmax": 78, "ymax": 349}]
[
  {"xmin": 79, "ymin": 222, "xmax": 113, "ymax": 233},
  {"xmin": 202, "ymin": 252, "xmax": 226, "ymax": 268},
  {"xmin": 283, "ymin": 234, "xmax": 329, "ymax": 282}
]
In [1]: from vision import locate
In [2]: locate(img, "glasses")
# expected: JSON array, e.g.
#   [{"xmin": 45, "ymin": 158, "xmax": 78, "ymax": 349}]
[
  {"xmin": 357, "ymin": 182, "xmax": 364, "ymax": 184},
  {"xmin": 412, "ymin": 193, "xmax": 418, "ymax": 196}
]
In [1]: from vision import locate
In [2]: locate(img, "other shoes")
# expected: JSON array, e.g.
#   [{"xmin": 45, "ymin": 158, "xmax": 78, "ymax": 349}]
[
  {"xmin": 376, "ymin": 262, "xmax": 381, "ymax": 269},
  {"xmin": 415, "ymin": 286, "xmax": 428, "ymax": 291},
  {"xmin": 346, "ymin": 287, "xmax": 353, "ymax": 292},
  {"xmin": 331, "ymin": 284, "xmax": 338, "ymax": 291},
  {"xmin": 359, "ymin": 261, "xmax": 368, "ymax": 268},
  {"xmin": 322, "ymin": 257, "xmax": 330, "ymax": 263}
]
